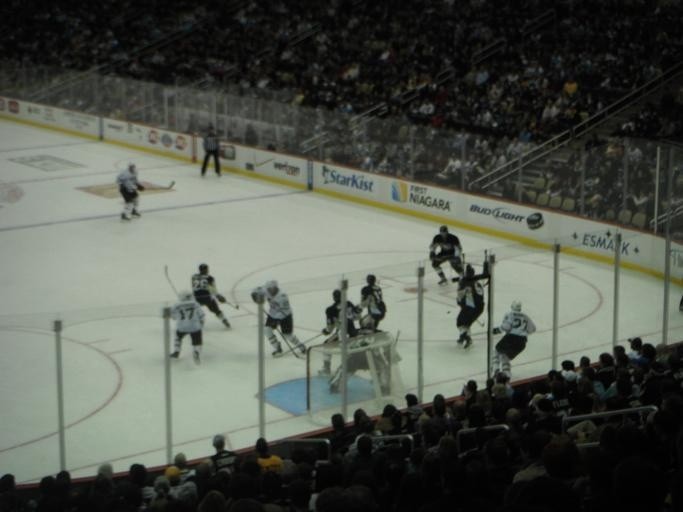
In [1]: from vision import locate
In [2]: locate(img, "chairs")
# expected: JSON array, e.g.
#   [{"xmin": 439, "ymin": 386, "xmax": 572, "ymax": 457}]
[{"xmin": 524, "ymin": 134, "xmax": 682, "ymax": 231}]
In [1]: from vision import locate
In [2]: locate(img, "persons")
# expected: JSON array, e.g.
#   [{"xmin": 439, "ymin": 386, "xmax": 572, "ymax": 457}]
[
  {"xmin": 191, "ymin": 263, "xmax": 230, "ymax": 328},
  {"xmin": 170, "ymin": 294, "xmax": 205, "ymax": 363},
  {"xmin": 356, "ymin": 275, "xmax": 386, "ymax": 335},
  {"xmin": 456, "ymin": 261, "xmax": 491, "ymax": 349},
  {"xmin": 492, "ymin": 300, "xmax": 536, "ymax": 381},
  {"xmin": 429, "ymin": 225, "xmax": 462, "ymax": 285},
  {"xmin": 251, "ymin": 280, "xmax": 306, "ymax": 356},
  {"xmin": 318, "ymin": 290, "xmax": 358, "ymax": 374}
]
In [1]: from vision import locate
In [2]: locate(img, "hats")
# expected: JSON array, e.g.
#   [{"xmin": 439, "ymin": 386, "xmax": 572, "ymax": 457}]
[{"xmin": 152, "ymin": 435, "xmax": 226, "ymax": 491}]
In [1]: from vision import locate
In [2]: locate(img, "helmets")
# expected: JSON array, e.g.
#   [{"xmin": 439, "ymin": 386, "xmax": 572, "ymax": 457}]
[{"xmin": 127, "ymin": 164, "xmax": 135, "ymax": 171}]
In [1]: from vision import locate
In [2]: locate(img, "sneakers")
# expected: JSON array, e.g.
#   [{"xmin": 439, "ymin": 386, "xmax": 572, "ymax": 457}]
[
  {"xmin": 438, "ymin": 276, "xmax": 461, "ymax": 286},
  {"xmin": 167, "ymin": 350, "xmax": 202, "ymax": 362},
  {"xmin": 456, "ymin": 335, "xmax": 474, "ymax": 347},
  {"xmin": 271, "ymin": 343, "xmax": 308, "ymax": 358},
  {"xmin": 319, "ymin": 369, "xmax": 330, "ymax": 376},
  {"xmin": 221, "ymin": 320, "xmax": 230, "ymax": 327},
  {"xmin": 119, "ymin": 209, "xmax": 141, "ymax": 218}
]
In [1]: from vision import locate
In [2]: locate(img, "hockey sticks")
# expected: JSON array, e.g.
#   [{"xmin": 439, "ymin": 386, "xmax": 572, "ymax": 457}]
[
  {"xmin": 477, "ymin": 318, "xmax": 485, "ymax": 326},
  {"xmin": 145, "ymin": 181, "xmax": 175, "ymax": 190},
  {"xmin": 263, "ymin": 307, "xmax": 306, "ymax": 360},
  {"xmin": 226, "ymin": 300, "xmax": 240, "ymax": 309},
  {"xmin": 273, "ymin": 332, "xmax": 323, "ymax": 359},
  {"xmin": 165, "ymin": 265, "xmax": 179, "ymax": 295}
]
